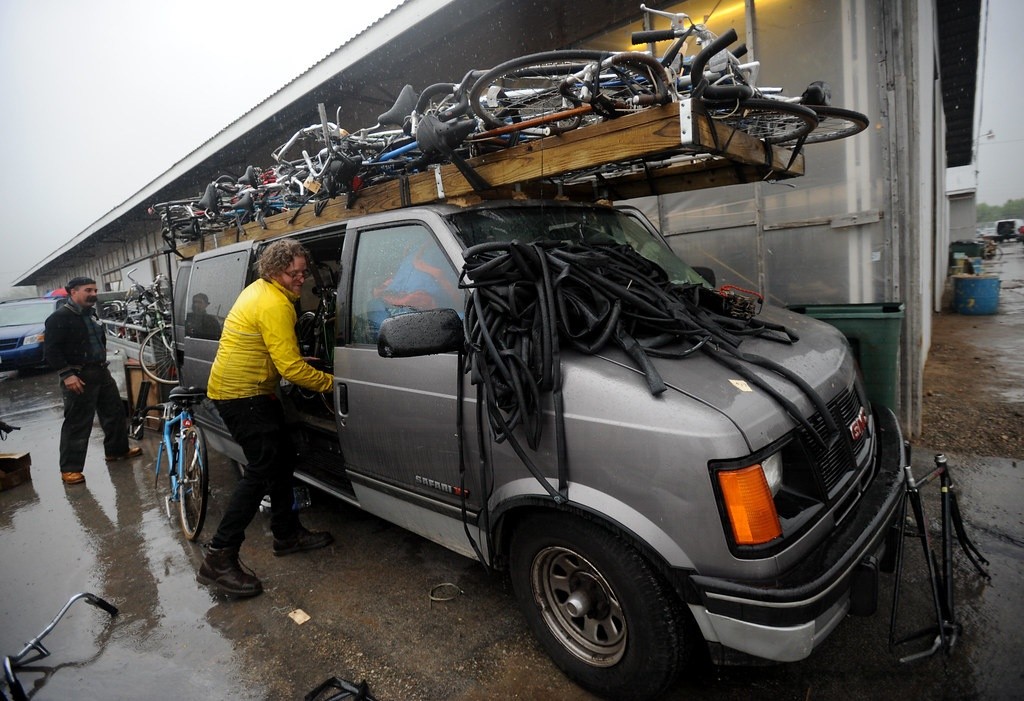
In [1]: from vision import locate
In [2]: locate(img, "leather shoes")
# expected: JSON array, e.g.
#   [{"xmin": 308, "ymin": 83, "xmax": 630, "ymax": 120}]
[
  {"xmin": 105, "ymin": 446, "xmax": 142, "ymax": 461},
  {"xmin": 62, "ymin": 471, "xmax": 85, "ymax": 484},
  {"xmin": 196, "ymin": 542, "xmax": 262, "ymax": 597},
  {"xmin": 272, "ymin": 525, "xmax": 335, "ymax": 556}
]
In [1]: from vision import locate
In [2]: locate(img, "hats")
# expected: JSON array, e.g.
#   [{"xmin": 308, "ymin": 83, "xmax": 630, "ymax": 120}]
[
  {"xmin": 193, "ymin": 292, "xmax": 210, "ymax": 305},
  {"xmin": 65, "ymin": 276, "xmax": 96, "ymax": 293}
]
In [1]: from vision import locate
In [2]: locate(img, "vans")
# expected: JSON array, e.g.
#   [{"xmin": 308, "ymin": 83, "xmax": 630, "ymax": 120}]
[
  {"xmin": 170, "ymin": 197, "xmax": 908, "ymax": 699},
  {"xmin": 975, "ymin": 219, "xmax": 1024, "ymax": 245}
]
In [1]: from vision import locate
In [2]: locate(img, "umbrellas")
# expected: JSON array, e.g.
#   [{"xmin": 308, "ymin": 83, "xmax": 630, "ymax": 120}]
[{"xmin": 45, "ymin": 289, "xmax": 68, "ymax": 298}]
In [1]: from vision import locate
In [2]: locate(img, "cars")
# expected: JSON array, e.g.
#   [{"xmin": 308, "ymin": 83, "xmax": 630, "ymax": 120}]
[{"xmin": 0, "ymin": 296, "xmax": 70, "ymax": 375}]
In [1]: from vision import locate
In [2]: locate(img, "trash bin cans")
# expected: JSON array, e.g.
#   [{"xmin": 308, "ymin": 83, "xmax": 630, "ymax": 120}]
[
  {"xmin": 949, "ymin": 239, "xmax": 985, "ymax": 274},
  {"xmin": 780, "ymin": 300, "xmax": 907, "ymax": 436}
]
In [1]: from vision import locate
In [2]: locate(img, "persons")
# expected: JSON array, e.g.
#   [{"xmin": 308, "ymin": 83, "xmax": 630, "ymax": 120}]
[
  {"xmin": 188, "ymin": 293, "xmax": 221, "ymax": 341},
  {"xmin": 197, "ymin": 239, "xmax": 334, "ymax": 597},
  {"xmin": 45, "ymin": 276, "xmax": 143, "ymax": 484}
]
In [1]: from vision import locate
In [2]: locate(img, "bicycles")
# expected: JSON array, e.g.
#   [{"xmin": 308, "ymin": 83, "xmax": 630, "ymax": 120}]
[
  {"xmin": 977, "ymin": 238, "xmax": 1004, "ymax": 262},
  {"xmin": 131, "ymin": 384, "xmax": 210, "ymax": 540},
  {"xmin": 0, "ymin": 591, "xmax": 120, "ymax": 701},
  {"xmin": 143, "ymin": 1, "xmax": 870, "ymax": 256},
  {"xmin": 98, "ymin": 268, "xmax": 181, "ymax": 383}
]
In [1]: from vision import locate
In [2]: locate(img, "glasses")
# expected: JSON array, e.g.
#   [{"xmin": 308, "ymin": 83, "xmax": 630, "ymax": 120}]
[{"xmin": 283, "ymin": 269, "xmax": 312, "ymax": 279}]
